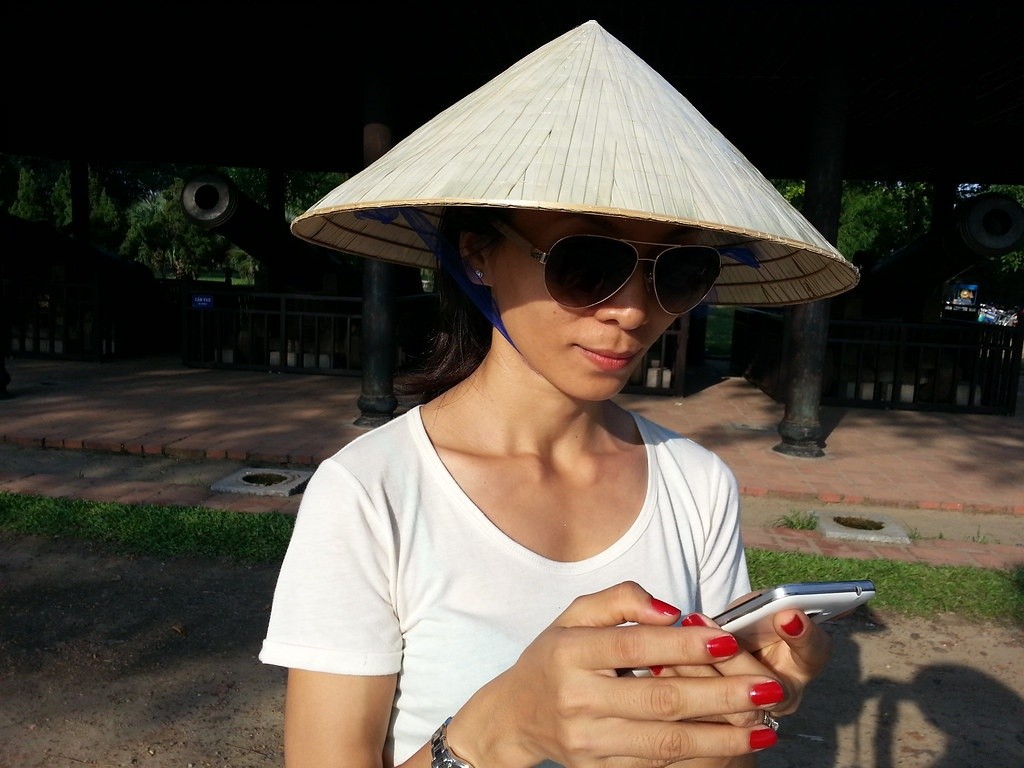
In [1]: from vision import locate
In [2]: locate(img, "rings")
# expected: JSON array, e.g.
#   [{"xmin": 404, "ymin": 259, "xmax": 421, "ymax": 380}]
[{"xmin": 763, "ymin": 711, "xmax": 779, "ymax": 731}]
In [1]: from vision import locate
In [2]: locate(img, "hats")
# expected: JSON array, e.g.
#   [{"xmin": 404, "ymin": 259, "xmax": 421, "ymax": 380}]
[{"xmin": 290, "ymin": 21, "xmax": 860, "ymax": 309}]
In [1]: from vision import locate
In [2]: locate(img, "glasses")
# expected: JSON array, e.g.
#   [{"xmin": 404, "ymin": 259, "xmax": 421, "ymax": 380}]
[{"xmin": 491, "ymin": 219, "xmax": 724, "ymax": 317}]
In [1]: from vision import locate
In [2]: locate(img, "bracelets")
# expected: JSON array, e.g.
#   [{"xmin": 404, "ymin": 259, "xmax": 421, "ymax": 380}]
[{"xmin": 431, "ymin": 716, "xmax": 471, "ymax": 768}]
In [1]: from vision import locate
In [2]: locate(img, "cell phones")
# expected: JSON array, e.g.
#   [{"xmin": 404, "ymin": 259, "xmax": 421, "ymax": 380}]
[{"xmin": 620, "ymin": 579, "xmax": 876, "ymax": 679}]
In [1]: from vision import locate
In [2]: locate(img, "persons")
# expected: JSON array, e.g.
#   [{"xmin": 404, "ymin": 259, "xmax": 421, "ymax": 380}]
[{"xmin": 257, "ymin": 19, "xmax": 861, "ymax": 768}]
